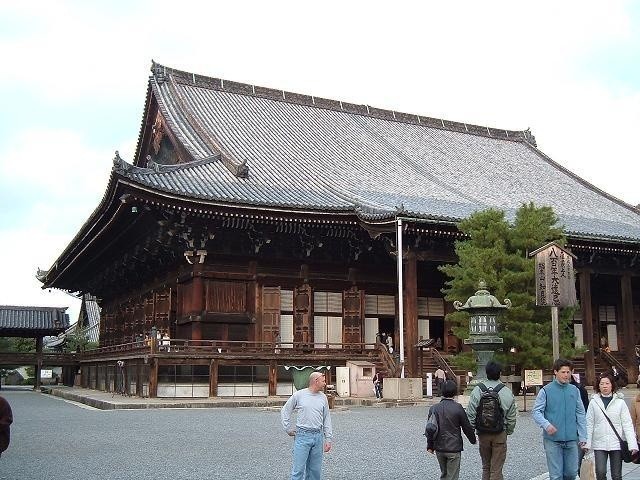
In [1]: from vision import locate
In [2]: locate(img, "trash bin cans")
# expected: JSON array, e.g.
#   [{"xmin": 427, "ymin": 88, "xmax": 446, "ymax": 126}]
[{"xmin": 74, "ymin": 373, "xmax": 81, "ymax": 385}]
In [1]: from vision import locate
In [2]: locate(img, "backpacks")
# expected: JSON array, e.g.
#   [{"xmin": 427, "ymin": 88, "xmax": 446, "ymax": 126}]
[{"xmin": 476, "ymin": 382, "xmax": 506, "ymax": 433}]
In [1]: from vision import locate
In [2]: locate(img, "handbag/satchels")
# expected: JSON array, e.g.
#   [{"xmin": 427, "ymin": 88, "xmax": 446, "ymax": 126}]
[
  {"xmin": 425, "ymin": 414, "xmax": 438, "ymax": 440},
  {"xmin": 620, "ymin": 441, "xmax": 629, "ymax": 463}
]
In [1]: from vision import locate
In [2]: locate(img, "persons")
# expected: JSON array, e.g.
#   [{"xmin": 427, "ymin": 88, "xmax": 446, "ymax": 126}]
[
  {"xmin": 280, "ymin": 371, "xmax": 333, "ymax": 480},
  {"xmin": 385, "ymin": 333, "xmax": 394, "ymax": 354},
  {"xmin": 426, "ymin": 379, "xmax": 478, "ymax": 480},
  {"xmin": 466, "ymin": 360, "xmax": 518, "ymax": 480},
  {"xmin": 380, "ymin": 331, "xmax": 388, "ymax": 344},
  {"xmin": 579, "ymin": 371, "xmax": 639, "ymax": 480},
  {"xmin": 0, "ymin": 395, "xmax": 14, "ymax": 458},
  {"xmin": 567, "ymin": 359, "xmax": 589, "ymax": 480},
  {"xmin": 373, "ymin": 371, "xmax": 383, "ymax": 399},
  {"xmin": 599, "ymin": 333, "xmax": 608, "ymax": 348},
  {"xmin": 629, "ymin": 374, "xmax": 640, "ymax": 466},
  {"xmin": 136, "ymin": 329, "xmax": 172, "ymax": 353},
  {"xmin": 610, "ymin": 364, "xmax": 620, "ymax": 387},
  {"xmin": 530, "ymin": 358, "xmax": 588, "ymax": 479},
  {"xmin": 434, "ymin": 366, "xmax": 447, "ymax": 397}
]
